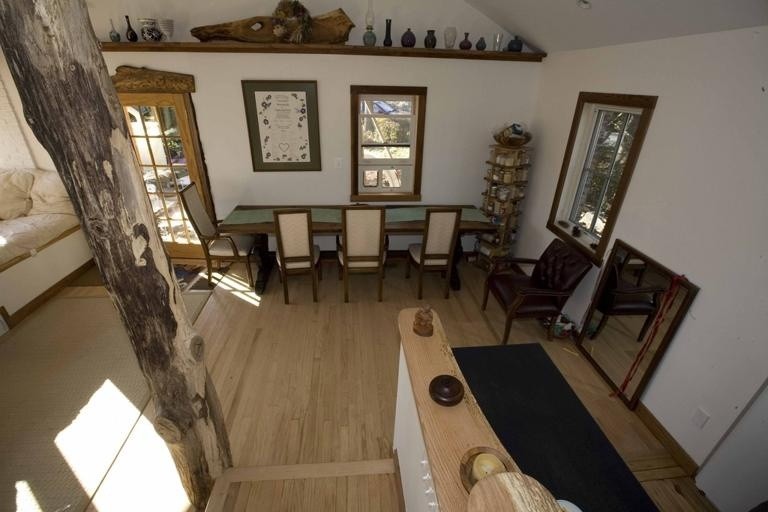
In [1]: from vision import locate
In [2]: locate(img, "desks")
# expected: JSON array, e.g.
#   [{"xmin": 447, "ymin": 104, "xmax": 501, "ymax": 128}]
[{"xmin": 217, "ymin": 197, "xmax": 480, "ymax": 292}]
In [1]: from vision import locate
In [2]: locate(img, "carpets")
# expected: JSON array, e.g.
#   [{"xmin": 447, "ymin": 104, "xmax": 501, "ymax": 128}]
[
  {"xmin": 69, "ymin": 258, "xmax": 207, "ymax": 293},
  {"xmin": 449, "ymin": 341, "xmax": 663, "ymax": 512}
]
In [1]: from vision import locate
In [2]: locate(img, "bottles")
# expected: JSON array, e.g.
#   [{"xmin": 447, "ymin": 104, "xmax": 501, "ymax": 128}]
[
  {"xmin": 401, "ymin": 28, "xmax": 417, "ymax": 48},
  {"xmin": 424, "ymin": 29, "xmax": 436, "ymax": 48},
  {"xmin": 508, "ymin": 35, "xmax": 522, "ymax": 52},
  {"xmin": 476, "ymin": 37, "xmax": 486, "ymax": 50},
  {"xmin": 459, "ymin": 32, "xmax": 472, "ymax": 49},
  {"xmin": 125, "ymin": 15, "xmax": 138, "ymax": 41},
  {"xmin": 383, "ymin": 19, "xmax": 392, "ymax": 47},
  {"xmin": 108, "ymin": 19, "xmax": 120, "ymax": 41}
]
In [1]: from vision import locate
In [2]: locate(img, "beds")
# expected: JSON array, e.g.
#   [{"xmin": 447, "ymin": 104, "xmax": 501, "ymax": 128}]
[{"xmin": 0, "ymin": 193, "xmax": 96, "ymax": 328}]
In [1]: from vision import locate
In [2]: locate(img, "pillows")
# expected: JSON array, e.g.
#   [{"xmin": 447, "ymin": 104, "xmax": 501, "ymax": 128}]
[{"xmin": 0, "ymin": 165, "xmax": 79, "ymax": 220}]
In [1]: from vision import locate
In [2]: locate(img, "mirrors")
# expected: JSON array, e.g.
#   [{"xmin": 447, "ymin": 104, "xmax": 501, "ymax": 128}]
[{"xmin": 577, "ymin": 237, "xmax": 702, "ymax": 412}]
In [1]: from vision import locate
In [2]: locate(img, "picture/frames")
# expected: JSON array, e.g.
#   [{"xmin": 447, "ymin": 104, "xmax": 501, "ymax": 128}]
[{"xmin": 238, "ymin": 79, "xmax": 324, "ymax": 173}]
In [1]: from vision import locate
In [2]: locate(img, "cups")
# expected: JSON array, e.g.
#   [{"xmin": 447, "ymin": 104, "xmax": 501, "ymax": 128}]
[
  {"xmin": 493, "ymin": 32, "xmax": 503, "ymax": 52},
  {"xmin": 444, "ymin": 28, "xmax": 456, "ymax": 49}
]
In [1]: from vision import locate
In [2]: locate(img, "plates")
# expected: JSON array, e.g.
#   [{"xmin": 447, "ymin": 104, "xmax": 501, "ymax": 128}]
[{"xmin": 460, "ymin": 446, "xmax": 516, "ymax": 494}]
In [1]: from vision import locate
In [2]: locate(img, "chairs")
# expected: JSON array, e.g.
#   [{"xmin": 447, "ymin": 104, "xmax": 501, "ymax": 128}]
[
  {"xmin": 480, "ymin": 237, "xmax": 594, "ymax": 343},
  {"xmin": 174, "ymin": 182, "xmax": 462, "ymax": 301},
  {"xmin": 587, "ymin": 258, "xmax": 669, "ymax": 344}
]
